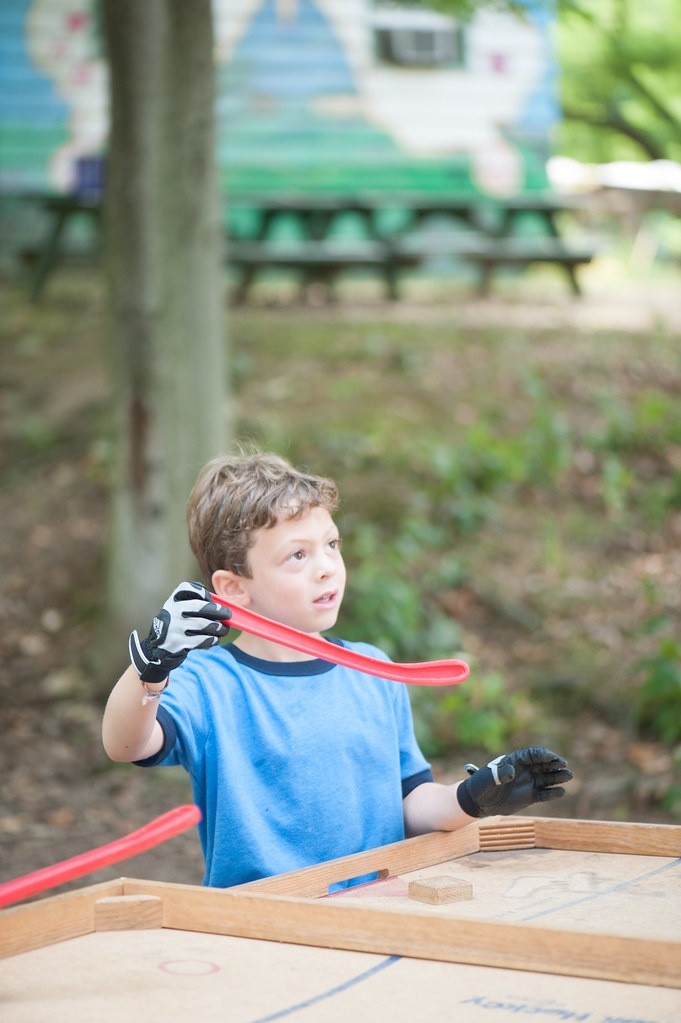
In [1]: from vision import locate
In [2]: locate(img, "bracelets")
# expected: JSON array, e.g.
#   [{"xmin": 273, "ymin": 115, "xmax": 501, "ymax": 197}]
[{"xmin": 142, "ymin": 677, "xmax": 169, "ymax": 706}]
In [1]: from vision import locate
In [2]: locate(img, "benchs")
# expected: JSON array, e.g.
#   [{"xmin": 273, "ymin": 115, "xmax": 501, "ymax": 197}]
[
  {"xmin": 225, "ymin": 253, "xmax": 422, "ymax": 282},
  {"xmin": 464, "ymin": 245, "xmax": 593, "ymax": 272}
]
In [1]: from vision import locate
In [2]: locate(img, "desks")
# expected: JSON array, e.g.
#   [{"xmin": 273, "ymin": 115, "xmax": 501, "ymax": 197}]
[
  {"xmin": 230, "ymin": 199, "xmax": 400, "ymax": 305},
  {"xmin": 404, "ymin": 199, "xmax": 583, "ymax": 298},
  {"xmin": 28, "ymin": 191, "xmax": 100, "ymax": 302},
  {"xmin": 0, "ymin": 848, "xmax": 681, "ymax": 1023}
]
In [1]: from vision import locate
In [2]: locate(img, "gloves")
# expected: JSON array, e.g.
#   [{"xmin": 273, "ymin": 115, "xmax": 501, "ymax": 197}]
[
  {"xmin": 128, "ymin": 579, "xmax": 231, "ymax": 683},
  {"xmin": 457, "ymin": 747, "xmax": 573, "ymax": 818}
]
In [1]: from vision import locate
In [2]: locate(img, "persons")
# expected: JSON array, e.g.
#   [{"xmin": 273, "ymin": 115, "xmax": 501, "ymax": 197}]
[{"xmin": 102, "ymin": 452, "xmax": 574, "ymax": 893}]
